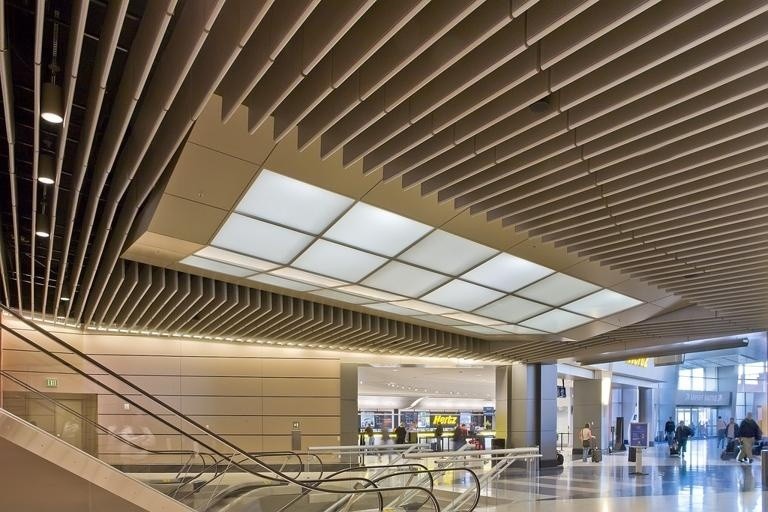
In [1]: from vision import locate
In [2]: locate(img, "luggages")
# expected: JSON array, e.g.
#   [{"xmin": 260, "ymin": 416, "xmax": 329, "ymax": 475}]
[
  {"xmin": 670, "ymin": 446, "xmax": 680, "ymax": 455},
  {"xmin": 592, "ymin": 444, "xmax": 602, "ymax": 463},
  {"xmin": 721, "ymin": 446, "xmax": 740, "ymax": 460}
]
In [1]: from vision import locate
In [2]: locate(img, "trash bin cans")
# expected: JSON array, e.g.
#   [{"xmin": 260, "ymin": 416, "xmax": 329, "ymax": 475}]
[{"xmin": 490, "ymin": 436, "xmax": 506, "ymax": 467}]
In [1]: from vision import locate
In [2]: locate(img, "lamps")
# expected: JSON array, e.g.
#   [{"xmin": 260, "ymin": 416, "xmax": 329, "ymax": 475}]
[
  {"xmin": 36, "ymin": 214, "xmax": 50, "ymax": 238},
  {"xmin": 36, "ymin": 153, "xmax": 57, "ymax": 185},
  {"xmin": 40, "ymin": 64, "xmax": 64, "ymax": 126}
]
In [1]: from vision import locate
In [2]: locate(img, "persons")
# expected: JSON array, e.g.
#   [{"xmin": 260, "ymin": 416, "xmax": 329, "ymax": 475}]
[
  {"xmin": 675, "ymin": 420, "xmax": 694, "ymax": 456},
  {"xmin": 664, "ymin": 417, "xmax": 676, "ymax": 446},
  {"xmin": 461, "ymin": 424, "xmax": 466, "ymax": 432},
  {"xmin": 734, "ymin": 411, "xmax": 762, "ymax": 463},
  {"xmin": 724, "ymin": 417, "xmax": 740, "ymax": 451},
  {"xmin": 716, "ymin": 416, "xmax": 728, "ymax": 449},
  {"xmin": 435, "ymin": 422, "xmax": 443, "ymax": 451},
  {"xmin": 395, "ymin": 422, "xmax": 407, "ymax": 444},
  {"xmin": 365, "ymin": 424, "xmax": 375, "ymax": 445},
  {"xmin": 412, "ymin": 422, "xmax": 417, "ymax": 428},
  {"xmin": 578, "ymin": 423, "xmax": 593, "ymax": 462}
]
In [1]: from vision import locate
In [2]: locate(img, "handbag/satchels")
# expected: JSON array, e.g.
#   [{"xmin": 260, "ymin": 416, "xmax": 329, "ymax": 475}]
[
  {"xmin": 583, "ymin": 440, "xmax": 590, "ymax": 447},
  {"xmin": 726, "ymin": 440, "xmax": 738, "ymax": 452}
]
